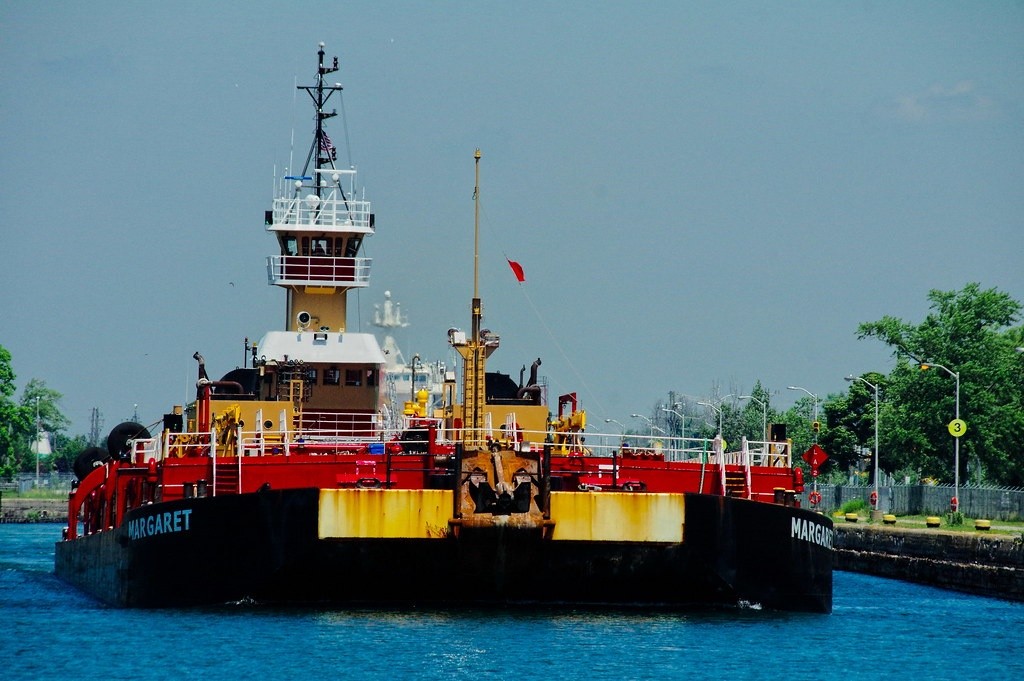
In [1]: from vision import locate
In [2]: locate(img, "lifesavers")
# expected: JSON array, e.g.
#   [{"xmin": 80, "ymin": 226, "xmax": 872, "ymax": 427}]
[
  {"xmin": 73, "ymin": 447, "xmax": 108, "ymax": 480},
  {"xmin": 950, "ymin": 496, "xmax": 957, "ymax": 511},
  {"xmin": 869, "ymin": 491, "xmax": 878, "ymax": 505},
  {"xmin": 401, "ymin": 425, "xmax": 429, "ymax": 454},
  {"xmin": 808, "ymin": 491, "xmax": 821, "ymax": 504},
  {"xmin": 622, "ymin": 481, "xmax": 646, "ymax": 492},
  {"xmin": 106, "ymin": 421, "xmax": 153, "ymax": 464}
]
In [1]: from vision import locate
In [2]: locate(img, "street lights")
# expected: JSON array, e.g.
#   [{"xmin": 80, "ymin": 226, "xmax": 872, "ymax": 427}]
[
  {"xmin": 843, "ymin": 373, "xmax": 883, "ymax": 522},
  {"xmin": 585, "ymin": 421, "xmax": 600, "ymax": 457},
  {"xmin": 35, "ymin": 395, "xmax": 44, "ymax": 492},
  {"xmin": 696, "ymin": 400, "xmax": 722, "ymax": 437},
  {"xmin": 604, "ymin": 418, "xmax": 625, "ymax": 445},
  {"xmin": 920, "ymin": 362, "xmax": 967, "ymax": 524},
  {"xmin": 737, "ymin": 395, "xmax": 766, "ymax": 467},
  {"xmin": 629, "ymin": 413, "xmax": 653, "ymax": 452},
  {"xmin": 786, "ymin": 385, "xmax": 829, "ymax": 515},
  {"xmin": 661, "ymin": 408, "xmax": 685, "ymax": 462}
]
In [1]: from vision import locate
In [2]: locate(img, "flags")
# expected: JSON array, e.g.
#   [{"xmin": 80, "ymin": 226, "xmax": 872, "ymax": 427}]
[{"xmin": 507, "ymin": 259, "xmax": 525, "ymax": 282}]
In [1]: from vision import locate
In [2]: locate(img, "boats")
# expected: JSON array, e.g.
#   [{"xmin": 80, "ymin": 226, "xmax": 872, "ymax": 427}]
[{"xmin": 54, "ymin": 38, "xmax": 834, "ymax": 617}]
